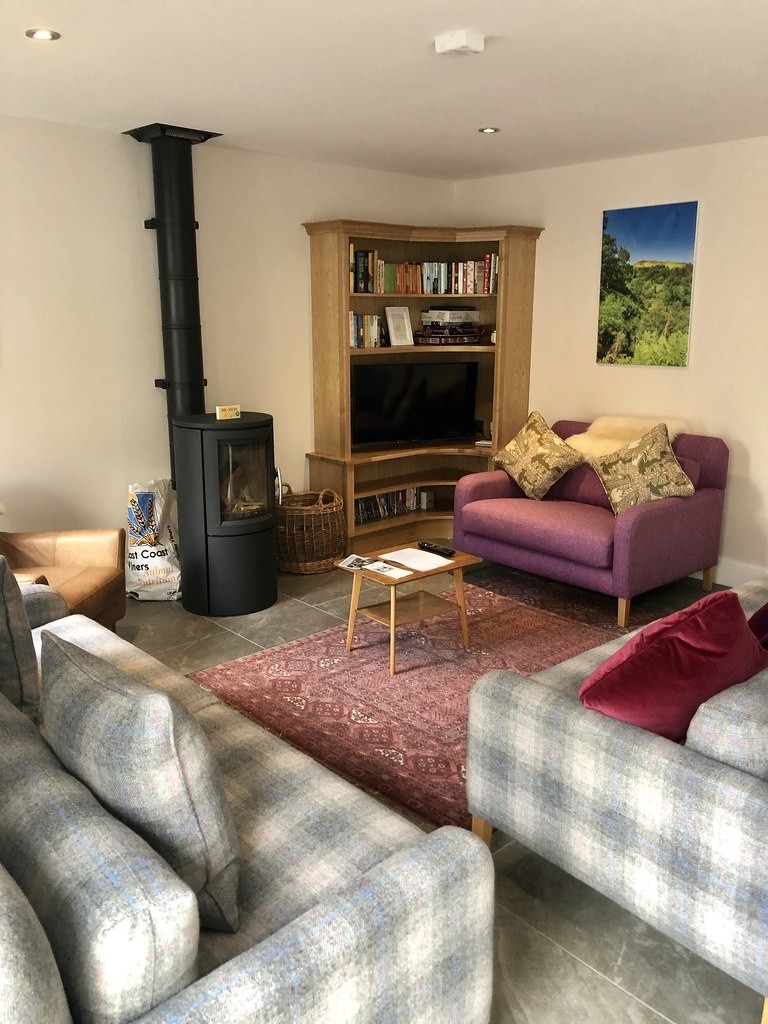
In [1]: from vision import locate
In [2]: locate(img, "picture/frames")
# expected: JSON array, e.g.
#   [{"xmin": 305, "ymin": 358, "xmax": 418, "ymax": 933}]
[{"xmin": 385, "ymin": 306, "xmax": 415, "ymax": 346}]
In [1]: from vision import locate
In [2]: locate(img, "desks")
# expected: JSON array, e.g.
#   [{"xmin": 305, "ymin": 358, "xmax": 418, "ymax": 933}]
[{"xmin": 333, "ymin": 541, "xmax": 483, "ymax": 677}]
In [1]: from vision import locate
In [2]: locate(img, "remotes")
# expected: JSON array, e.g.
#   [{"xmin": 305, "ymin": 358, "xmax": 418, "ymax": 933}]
[{"xmin": 418, "ymin": 541, "xmax": 456, "ymax": 558}]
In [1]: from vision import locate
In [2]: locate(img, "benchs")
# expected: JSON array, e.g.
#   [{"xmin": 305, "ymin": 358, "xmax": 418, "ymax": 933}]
[
  {"xmin": 452, "ymin": 420, "xmax": 729, "ymax": 627},
  {"xmin": 467, "ymin": 577, "xmax": 768, "ymax": 1024},
  {"xmin": 0, "ymin": 584, "xmax": 492, "ymax": 1024}
]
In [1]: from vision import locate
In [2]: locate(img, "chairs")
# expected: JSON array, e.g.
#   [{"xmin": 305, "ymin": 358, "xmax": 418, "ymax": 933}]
[{"xmin": 0, "ymin": 527, "xmax": 126, "ymax": 634}]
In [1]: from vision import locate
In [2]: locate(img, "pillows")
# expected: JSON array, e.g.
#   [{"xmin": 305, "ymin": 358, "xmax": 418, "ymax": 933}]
[
  {"xmin": 0, "ymin": 556, "xmax": 41, "ymax": 719},
  {"xmin": 577, "ymin": 590, "xmax": 768, "ymax": 745},
  {"xmin": 38, "ymin": 629, "xmax": 238, "ymax": 931},
  {"xmin": 491, "ymin": 411, "xmax": 584, "ymax": 499},
  {"xmin": 591, "ymin": 424, "xmax": 695, "ymax": 517}
]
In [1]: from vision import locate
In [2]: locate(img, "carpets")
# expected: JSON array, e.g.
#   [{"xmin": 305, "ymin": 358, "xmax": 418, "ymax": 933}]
[{"xmin": 182, "ymin": 569, "xmax": 732, "ymax": 832}]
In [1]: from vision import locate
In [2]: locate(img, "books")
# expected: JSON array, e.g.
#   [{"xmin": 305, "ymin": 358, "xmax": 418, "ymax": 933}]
[
  {"xmin": 348, "ymin": 243, "xmax": 498, "ymax": 295},
  {"xmin": 353, "ymin": 486, "xmax": 421, "ymax": 525},
  {"xmin": 348, "ymin": 310, "xmax": 382, "ymax": 348}
]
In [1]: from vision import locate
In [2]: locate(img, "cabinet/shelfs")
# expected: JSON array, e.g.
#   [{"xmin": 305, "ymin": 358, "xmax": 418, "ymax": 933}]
[{"xmin": 302, "ymin": 219, "xmax": 546, "ymax": 552}]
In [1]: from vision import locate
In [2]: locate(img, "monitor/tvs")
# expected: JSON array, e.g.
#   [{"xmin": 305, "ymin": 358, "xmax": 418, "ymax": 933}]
[{"xmin": 352, "ymin": 361, "xmax": 478, "ymax": 452}]
